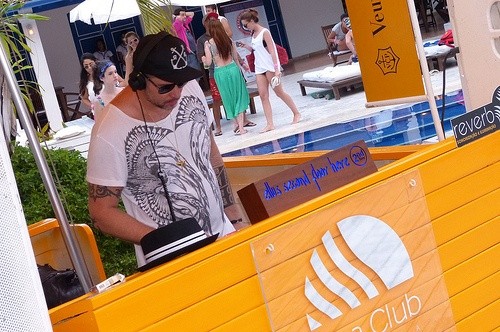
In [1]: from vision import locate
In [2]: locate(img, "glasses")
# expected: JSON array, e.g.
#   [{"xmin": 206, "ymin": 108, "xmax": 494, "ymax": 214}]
[
  {"xmin": 142, "ymin": 72, "xmax": 186, "ymax": 94},
  {"xmin": 244, "ymin": 22, "xmax": 248, "ymax": 28},
  {"xmin": 129, "ymin": 39, "xmax": 137, "ymax": 45},
  {"xmin": 84, "ymin": 64, "xmax": 93, "ymax": 69}
]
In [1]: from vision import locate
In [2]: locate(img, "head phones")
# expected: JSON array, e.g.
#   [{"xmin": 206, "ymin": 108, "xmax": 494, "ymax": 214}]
[{"xmin": 128, "ymin": 31, "xmax": 169, "ymax": 90}]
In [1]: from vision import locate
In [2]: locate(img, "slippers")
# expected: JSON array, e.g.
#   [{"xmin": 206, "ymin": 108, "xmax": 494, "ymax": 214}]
[
  {"xmin": 215, "ymin": 131, "xmax": 222, "ymax": 136},
  {"xmin": 244, "ymin": 122, "xmax": 257, "ymax": 127}
]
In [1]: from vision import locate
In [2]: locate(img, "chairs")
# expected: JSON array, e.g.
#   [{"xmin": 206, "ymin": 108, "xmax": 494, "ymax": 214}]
[{"xmin": 321, "ymin": 24, "xmax": 352, "ymax": 67}]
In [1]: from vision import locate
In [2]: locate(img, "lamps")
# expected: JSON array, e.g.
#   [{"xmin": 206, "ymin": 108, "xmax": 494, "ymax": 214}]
[{"xmin": 26, "ymin": 24, "xmax": 34, "ymax": 35}]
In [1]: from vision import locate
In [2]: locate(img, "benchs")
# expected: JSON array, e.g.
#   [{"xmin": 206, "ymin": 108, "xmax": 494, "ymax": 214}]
[
  {"xmin": 204, "ymin": 88, "xmax": 260, "ymax": 119},
  {"xmin": 297, "ymin": 39, "xmax": 457, "ymax": 100}
]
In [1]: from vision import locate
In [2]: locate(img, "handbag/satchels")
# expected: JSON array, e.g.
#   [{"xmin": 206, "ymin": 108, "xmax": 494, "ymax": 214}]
[{"xmin": 246, "ymin": 49, "xmax": 255, "ymax": 72}]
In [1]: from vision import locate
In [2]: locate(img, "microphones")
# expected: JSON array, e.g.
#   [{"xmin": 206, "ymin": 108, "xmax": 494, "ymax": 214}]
[{"xmin": 158, "ymin": 172, "xmax": 176, "ymax": 222}]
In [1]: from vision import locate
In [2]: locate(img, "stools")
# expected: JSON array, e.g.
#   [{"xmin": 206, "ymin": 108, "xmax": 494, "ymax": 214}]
[{"xmin": 62, "ymin": 93, "xmax": 94, "ymax": 121}]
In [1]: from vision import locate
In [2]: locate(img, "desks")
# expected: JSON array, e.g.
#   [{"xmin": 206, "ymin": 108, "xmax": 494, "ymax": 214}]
[{"xmin": 23, "ymin": 86, "xmax": 70, "ymax": 132}]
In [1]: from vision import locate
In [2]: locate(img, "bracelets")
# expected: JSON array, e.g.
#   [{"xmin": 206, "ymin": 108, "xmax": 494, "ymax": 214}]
[{"xmin": 231, "ymin": 218, "xmax": 242, "ymax": 224}]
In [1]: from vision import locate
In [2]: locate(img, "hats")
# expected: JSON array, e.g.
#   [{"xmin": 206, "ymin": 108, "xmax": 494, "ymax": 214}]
[
  {"xmin": 202, "ymin": 12, "xmax": 218, "ymax": 26},
  {"xmin": 133, "ymin": 31, "xmax": 204, "ymax": 83},
  {"xmin": 137, "ymin": 217, "xmax": 220, "ymax": 272}
]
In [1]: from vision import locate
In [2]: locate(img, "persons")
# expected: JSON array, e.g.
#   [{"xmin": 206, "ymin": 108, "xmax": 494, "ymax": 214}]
[
  {"xmin": 196, "ymin": 12, "xmax": 257, "ymax": 136},
  {"xmin": 201, "ymin": 18, "xmax": 250, "ymax": 135},
  {"xmin": 172, "ymin": 8, "xmax": 203, "ymax": 84},
  {"xmin": 116, "ymin": 32, "xmax": 129, "ymax": 80},
  {"xmin": 86, "ymin": 31, "xmax": 246, "ymax": 268},
  {"xmin": 328, "ymin": 14, "xmax": 348, "ymax": 51},
  {"xmin": 241, "ymin": 148, "xmax": 252, "ymax": 155},
  {"xmin": 42, "ymin": 274, "xmax": 87, "ymax": 310},
  {"xmin": 80, "ymin": 52, "xmax": 101, "ymax": 121},
  {"xmin": 272, "ymin": 132, "xmax": 304, "ymax": 154},
  {"xmin": 125, "ymin": 32, "xmax": 140, "ymax": 85},
  {"xmin": 205, "ymin": 4, "xmax": 233, "ymax": 37},
  {"xmin": 350, "ymin": 109, "xmax": 393, "ymax": 144},
  {"xmin": 92, "ymin": 60, "xmax": 125, "ymax": 121},
  {"xmin": 238, "ymin": 9, "xmax": 301, "ymax": 133},
  {"xmin": 93, "ymin": 38, "xmax": 115, "ymax": 64},
  {"xmin": 344, "ymin": 18, "xmax": 359, "ymax": 65}
]
